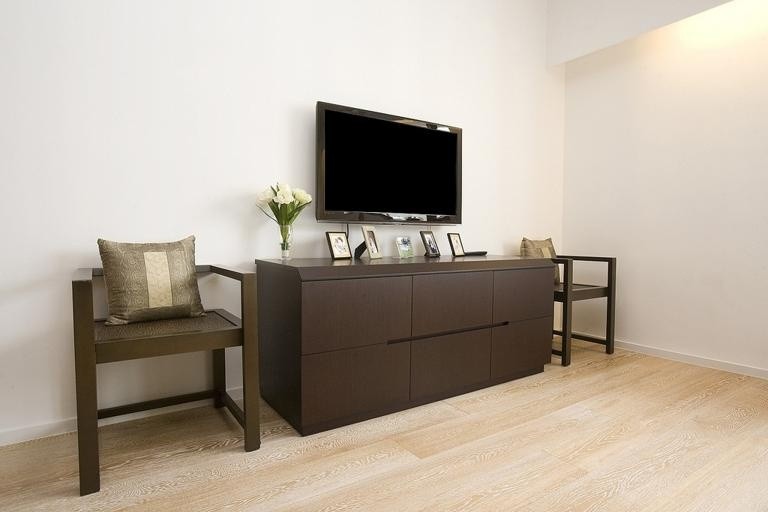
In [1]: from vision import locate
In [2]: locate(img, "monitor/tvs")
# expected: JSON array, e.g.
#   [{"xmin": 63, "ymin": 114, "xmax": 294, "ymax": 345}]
[{"xmin": 315, "ymin": 102, "xmax": 463, "ymax": 225}]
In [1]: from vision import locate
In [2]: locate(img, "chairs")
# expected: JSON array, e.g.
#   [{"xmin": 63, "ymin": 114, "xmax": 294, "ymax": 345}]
[
  {"xmin": 71, "ymin": 264, "xmax": 260, "ymax": 496},
  {"xmin": 552, "ymin": 254, "xmax": 618, "ymax": 367}
]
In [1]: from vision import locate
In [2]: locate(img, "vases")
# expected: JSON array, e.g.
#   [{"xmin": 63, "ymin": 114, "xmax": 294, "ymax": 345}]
[{"xmin": 275, "ymin": 224, "xmax": 294, "ymax": 260}]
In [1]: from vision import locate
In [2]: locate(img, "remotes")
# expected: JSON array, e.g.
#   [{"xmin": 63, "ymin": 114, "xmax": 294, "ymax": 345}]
[{"xmin": 464, "ymin": 250, "xmax": 487, "ymax": 257}]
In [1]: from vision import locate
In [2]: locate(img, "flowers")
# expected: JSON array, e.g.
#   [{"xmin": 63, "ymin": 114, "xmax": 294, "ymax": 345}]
[{"xmin": 255, "ymin": 184, "xmax": 314, "ymax": 249}]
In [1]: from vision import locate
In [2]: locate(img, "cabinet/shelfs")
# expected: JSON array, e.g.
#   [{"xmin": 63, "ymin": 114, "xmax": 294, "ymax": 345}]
[{"xmin": 256, "ymin": 254, "xmax": 556, "ymax": 437}]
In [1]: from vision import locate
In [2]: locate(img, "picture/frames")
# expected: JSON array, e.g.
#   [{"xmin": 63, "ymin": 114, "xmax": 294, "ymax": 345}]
[
  {"xmin": 326, "ymin": 231, "xmax": 353, "ymax": 261},
  {"xmin": 448, "ymin": 233, "xmax": 466, "ymax": 257},
  {"xmin": 361, "ymin": 225, "xmax": 382, "ymax": 259},
  {"xmin": 420, "ymin": 230, "xmax": 440, "ymax": 257},
  {"xmin": 394, "ymin": 235, "xmax": 415, "ymax": 258}
]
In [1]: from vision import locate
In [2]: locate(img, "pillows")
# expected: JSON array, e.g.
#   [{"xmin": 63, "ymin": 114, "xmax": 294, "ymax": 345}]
[
  {"xmin": 98, "ymin": 236, "xmax": 204, "ymax": 328},
  {"xmin": 521, "ymin": 236, "xmax": 560, "ymax": 284}
]
